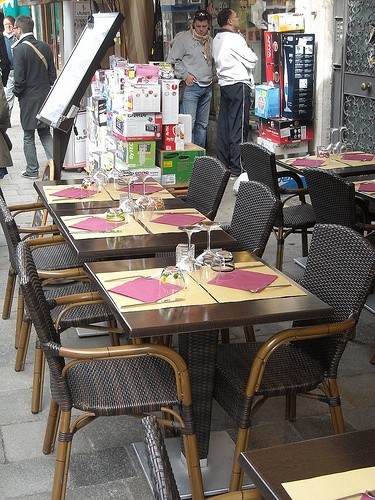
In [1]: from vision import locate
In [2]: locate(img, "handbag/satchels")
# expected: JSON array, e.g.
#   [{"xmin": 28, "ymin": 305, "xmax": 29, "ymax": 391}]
[{"xmin": 1, "ymin": 130, "xmax": 12, "ymax": 151}]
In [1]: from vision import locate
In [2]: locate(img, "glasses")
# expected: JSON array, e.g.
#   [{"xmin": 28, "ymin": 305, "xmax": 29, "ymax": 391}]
[
  {"xmin": 228, "ymin": 15, "xmax": 239, "ymax": 19},
  {"xmin": 195, "ymin": 13, "xmax": 209, "ymax": 17},
  {"xmin": 12, "ymin": 25, "xmax": 19, "ymax": 30}
]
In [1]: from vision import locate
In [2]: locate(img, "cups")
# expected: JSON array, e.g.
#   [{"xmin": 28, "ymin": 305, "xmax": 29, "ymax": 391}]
[
  {"xmin": 317, "ymin": 146, "xmax": 330, "ymax": 164},
  {"xmin": 159, "ymin": 244, "xmax": 235, "ymax": 305},
  {"xmin": 81, "ymin": 177, "xmax": 100, "ymax": 197},
  {"xmin": 104, "ymin": 194, "xmax": 165, "ymax": 226}
]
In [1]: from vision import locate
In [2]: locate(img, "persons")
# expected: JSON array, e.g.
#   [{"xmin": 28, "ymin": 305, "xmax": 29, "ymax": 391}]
[
  {"xmin": 167, "ymin": 10, "xmax": 218, "ymax": 149},
  {"xmin": 211, "ymin": 8, "xmax": 259, "ymax": 175},
  {"xmin": 11, "ymin": 15, "xmax": 57, "ymax": 179},
  {"xmin": 0, "ymin": 16, "xmax": 16, "ymax": 179}
]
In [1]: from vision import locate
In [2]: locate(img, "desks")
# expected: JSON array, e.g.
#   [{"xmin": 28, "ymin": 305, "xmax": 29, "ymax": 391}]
[
  {"xmin": 238, "ymin": 428, "xmax": 375, "ymax": 500},
  {"xmin": 83, "ymin": 249, "xmax": 334, "ymax": 500},
  {"xmin": 34, "ymin": 175, "xmax": 184, "ymax": 216},
  {"xmin": 52, "ymin": 203, "xmax": 237, "ymax": 261},
  {"xmin": 344, "ymin": 173, "xmax": 375, "ymax": 218},
  {"xmin": 276, "ymin": 150, "xmax": 375, "ymax": 178}
]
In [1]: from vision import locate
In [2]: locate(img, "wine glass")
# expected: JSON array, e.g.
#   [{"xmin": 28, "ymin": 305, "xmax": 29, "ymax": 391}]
[
  {"xmin": 90, "ymin": 150, "xmax": 121, "ymax": 187},
  {"xmin": 118, "ymin": 171, "xmax": 157, "ymax": 218},
  {"xmin": 175, "ymin": 220, "xmax": 225, "ymax": 284},
  {"xmin": 326, "ymin": 127, "xmax": 354, "ymax": 161}
]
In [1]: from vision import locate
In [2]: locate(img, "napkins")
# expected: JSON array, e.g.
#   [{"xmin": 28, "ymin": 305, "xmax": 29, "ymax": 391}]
[
  {"xmin": 49, "ymin": 187, "xmax": 98, "ymax": 200},
  {"xmin": 207, "ymin": 269, "xmax": 278, "ymax": 293},
  {"xmin": 68, "ymin": 216, "xmax": 126, "ymax": 233},
  {"xmin": 358, "ymin": 183, "xmax": 375, "ymax": 192},
  {"xmin": 106, "ymin": 277, "xmax": 184, "ymax": 304},
  {"xmin": 150, "ymin": 213, "xmax": 206, "ymax": 228},
  {"xmin": 289, "ymin": 158, "xmax": 325, "ymax": 168},
  {"xmin": 340, "ymin": 154, "xmax": 374, "ymax": 161},
  {"xmin": 116, "ymin": 184, "xmax": 164, "ymax": 196}
]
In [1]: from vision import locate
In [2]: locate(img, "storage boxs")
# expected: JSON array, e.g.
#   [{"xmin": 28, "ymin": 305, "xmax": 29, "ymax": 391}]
[
  {"xmin": 253, "ymin": 13, "xmax": 314, "ymax": 160},
  {"xmin": 84, "ymin": 56, "xmax": 206, "ymax": 187}
]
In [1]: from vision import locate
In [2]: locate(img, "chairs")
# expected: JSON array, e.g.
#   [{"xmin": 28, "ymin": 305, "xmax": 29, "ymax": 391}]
[{"xmin": 0, "ymin": 141, "xmax": 375, "ymax": 500}]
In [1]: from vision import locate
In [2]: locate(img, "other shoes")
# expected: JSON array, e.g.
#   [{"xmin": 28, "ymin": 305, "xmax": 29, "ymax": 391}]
[
  {"xmin": 22, "ymin": 172, "xmax": 38, "ymax": 179},
  {"xmin": 230, "ymin": 173, "xmax": 241, "ymax": 180}
]
